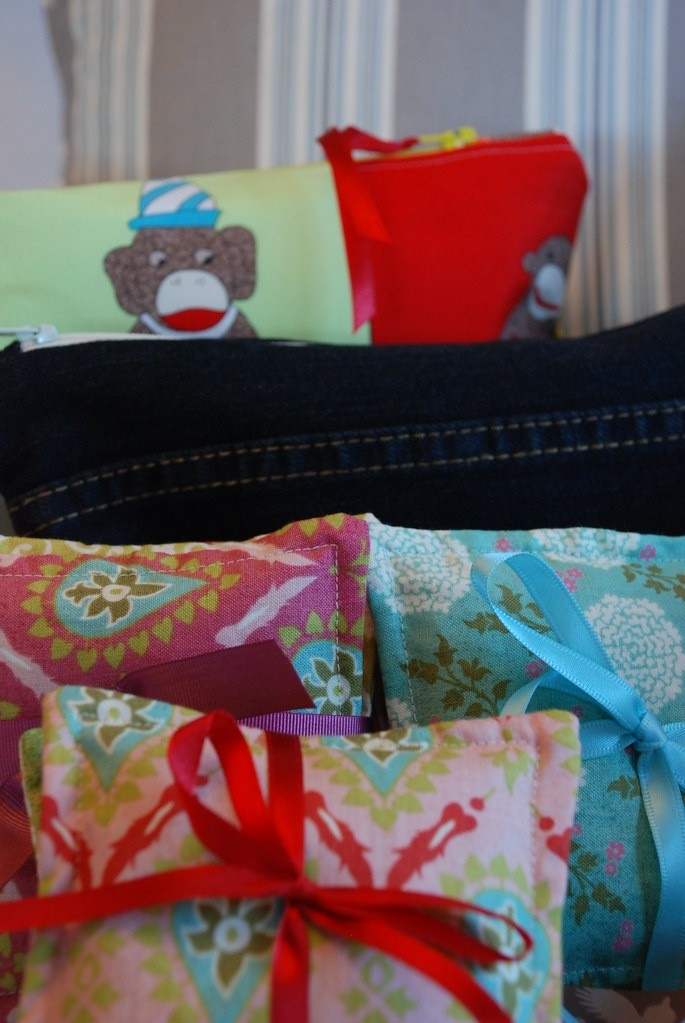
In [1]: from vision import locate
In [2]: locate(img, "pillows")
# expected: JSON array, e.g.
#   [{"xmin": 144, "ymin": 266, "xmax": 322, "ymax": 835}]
[{"xmin": 0, "ymin": 508, "xmax": 685, "ymax": 1023}]
